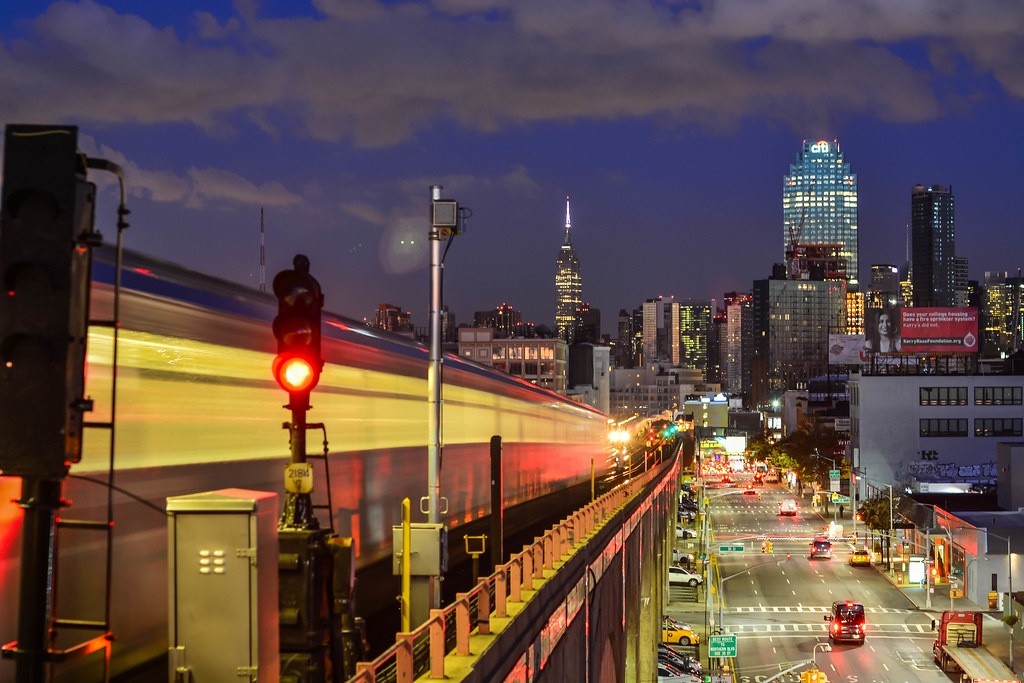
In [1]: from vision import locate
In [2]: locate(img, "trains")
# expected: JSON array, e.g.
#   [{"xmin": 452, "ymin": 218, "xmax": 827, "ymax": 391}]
[{"xmin": 1, "ymin": 238, "xmax": 629, "ymax": 683}]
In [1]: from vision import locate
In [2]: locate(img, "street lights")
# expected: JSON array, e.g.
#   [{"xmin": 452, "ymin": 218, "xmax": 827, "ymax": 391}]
[
  {"xmin": 966, "ymin": 557, "xmax": 989, "ymax": 600},
  {"xmin": 425, "ymin": 184, "xmax": 468, "ymax": 607},
  {"xmin": 917, "ymin": 503, "xmax": 954, "ymax": 613},
  {"xmin": 720, "ymin": 556, "xmax": 788, "ymax": 668},
  {"xmin": 855, "ymin": 475, "xmax": 894, "ymax": 578},
  {"xmin": 810, "ymin": 453, "xmax": 837, "ymax": 524},
  {"xmin": 955, "ymin": 525, "xmax": 1014, "ymax": 672},
  {"xmin": 709, "ymin": 535, "xmax": 769, "ymax": 625}
]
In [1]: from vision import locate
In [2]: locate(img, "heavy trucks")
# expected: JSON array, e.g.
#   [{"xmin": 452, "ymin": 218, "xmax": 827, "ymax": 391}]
[{"xmin": 931, "ymin": 609, "xmax": 1021, "ymax": 683}]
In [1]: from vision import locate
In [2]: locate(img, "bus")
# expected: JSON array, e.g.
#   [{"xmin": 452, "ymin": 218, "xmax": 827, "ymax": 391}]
[{"xmin": 755, "ymin": 462, "xmax": 766, "ymax": 477}]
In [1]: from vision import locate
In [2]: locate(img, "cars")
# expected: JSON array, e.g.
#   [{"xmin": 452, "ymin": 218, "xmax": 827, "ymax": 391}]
[
  {"xmin": 673, "ymin": 547, "xmax": 694, "ymax": 563},
  {"xmin": 675, "ymin": 525, "xmax": 697, "ymax": 538},
  {"xmin": 744, "ymin": 483, "xmax": 755, "ymax": 495},
  {"xmin": 704, "ymin": 473, "xmax": 740, "ymax": 488},
  {"xmin": 676, "ymin": 483, "xmax": 698, "ymax": 523},
  {"xmin": 778, "ymin": 499, "xmax": 798, "ymax": 516},
  {"xmin": 808, "ymin": 538, "xmax": 833, "ymax": 560},
  {"xmin": 848, "ymin": 550, "xmax": 871, "ymax": 567},
  {"xmin": 657, "ymin": 615, "xmax": 704, "ymax": 683}
]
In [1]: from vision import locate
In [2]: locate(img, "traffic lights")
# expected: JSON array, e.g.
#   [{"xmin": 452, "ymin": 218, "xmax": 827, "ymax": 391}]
[
  {"xmin": 799, "ymin": 670, "xmax": 810, "ymax": 683},
  {"xmin": 815, "ymin": 669, "xmax": 826, "ymax": 683},
  {"xmin": 270, "ymin": 268, "xmax": 323, "ymax": 397}
]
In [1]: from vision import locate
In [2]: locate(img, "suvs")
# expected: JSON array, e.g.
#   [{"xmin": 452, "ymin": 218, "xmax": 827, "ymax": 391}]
[
  {"xmin": 669, "ymin": 565, "xmax": 703, "ymax": 588},
  {"xmin": 823, "ymin": 600, "xmax": 866, "ymax": 644}
]
